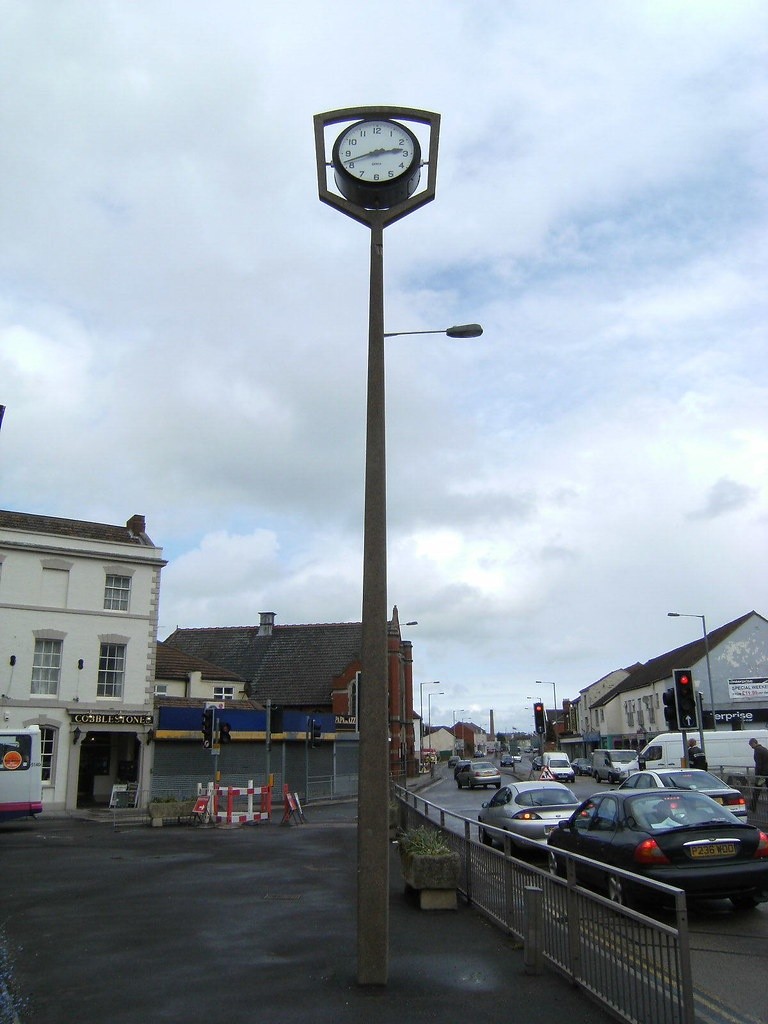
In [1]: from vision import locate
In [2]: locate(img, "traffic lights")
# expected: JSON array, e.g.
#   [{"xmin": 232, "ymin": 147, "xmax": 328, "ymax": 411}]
[
  {"xmin": 219, "ymin": 722, "xmax": 231, "ymax": 744},
  {"xmin": 310, "ymin": 720, "xmax": 321, "ymax": 749},
  {"xmin": 195, "ymin": 708, "xmax": 213, "ymax": 749},
  {"xmin": 533, "ymin": 703, "xmax": 547, "ymax": 734}
]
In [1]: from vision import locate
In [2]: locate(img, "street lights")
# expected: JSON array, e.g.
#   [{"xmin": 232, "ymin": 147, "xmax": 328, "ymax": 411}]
[
  {"xmin": 420, "ymin": 681, "xmax": 440, "ymax": 775},
  {"xmin": 667, "ymin": 612, "xmax": 717, "ymax": 730},
  {"xmin": 428, "ymin": 692, "xmax": 444, "ymax": 753},
  {"xmin": 352, "ymin": 324, "xmax": 484, "ymax": 990},
  {"xmin": 462, "ymin": 717, "xmax": 472, "ymax": 757},
  {"xmin": 536, "ymin": 681, "xmax": 559, "ymax": 752},
  {"xmin": 453, "ymin": 710, "xmax": 464, "ymax": 756},
  {"xmin": 526, "ymin": 696, "xmax": 544, "ymax": 754}
]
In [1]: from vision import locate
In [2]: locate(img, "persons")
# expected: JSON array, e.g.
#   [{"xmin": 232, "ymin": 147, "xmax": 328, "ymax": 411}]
[
  {"xmin": 746, "ymin": 738, "xmax": 768, "ymax": 813},
  {"xmin": 636, "ymin": 747, "xmax": 646, "ymax": 769},
  {"xmin": 687, "ymin": 739, "xmax": 705, "ymax": 770}
]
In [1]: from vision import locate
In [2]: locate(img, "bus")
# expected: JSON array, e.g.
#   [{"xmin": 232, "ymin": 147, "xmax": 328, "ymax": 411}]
[{"xmin": 0, "ymin": 725, "xmax": 43, "ymax": 822}]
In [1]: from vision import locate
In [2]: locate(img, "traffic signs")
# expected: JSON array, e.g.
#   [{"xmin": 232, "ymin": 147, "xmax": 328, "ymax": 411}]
[
  {"xmin": 662, "ymin": 687, "xmax": 678, "ymax": 732},
  {"xmin": 673, "ymin": 668, "xmax": 699, "ymax": 731}
]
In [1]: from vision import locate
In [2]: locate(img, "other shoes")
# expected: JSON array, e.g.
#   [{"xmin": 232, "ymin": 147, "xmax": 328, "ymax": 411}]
[{"xmin": 747, "ymin": 805, "xmax": 756, "ymax": 813}]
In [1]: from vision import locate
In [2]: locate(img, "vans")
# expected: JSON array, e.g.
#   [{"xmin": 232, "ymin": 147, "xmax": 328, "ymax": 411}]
[
  {"xmin": 618, "ymin": 730, "xmax": 768, "ymax": 785},
  {"xmin": 593, "ymin": 749, "xmax": 641, "ymax": 785}
]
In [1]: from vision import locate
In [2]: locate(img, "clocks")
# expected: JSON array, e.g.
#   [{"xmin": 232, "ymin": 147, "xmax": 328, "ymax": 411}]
[{"xmin": 331, "ymin": 116, "xmax": 422, "ymax": 210}]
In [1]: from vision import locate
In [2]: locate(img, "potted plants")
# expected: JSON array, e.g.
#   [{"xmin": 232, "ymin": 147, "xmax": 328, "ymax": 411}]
[
  {"xmin": 390, "ymin": 824, "xmax": 461, "ymax": 889},
  {"xmin": 148, "ymin": 795, "xmax": 200, "ymax": 818}
]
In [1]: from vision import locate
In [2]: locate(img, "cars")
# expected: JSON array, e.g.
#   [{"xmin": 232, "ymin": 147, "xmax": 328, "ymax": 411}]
[
  {"xmin": 524, "ymin": 746, "xmax": 540, "ymax": 754},
  {"xmin": 546, "ymin": 788, "xmax": 768, "ymax": 919},
  {"xmin": 531, "ymin": 757, "xmax": 542, "ymax": 771},
  {"xmin": 570, "ymin": 758, "xmax": 591, "ymax": 777},
  {"xmin": 474, "ymin": 752, "xmax": 484, "ymax": 758},
  {"xmin": 456, "ymin": 761, "xmax": 501, "ymax": 791},
  {"xmin": 453, "ymin": 760, "xmax": 472, "ymax": 780},
  {"xmin": 512, "ymin": 753, "xmax": 521, "ymax": 762},
  {"xmin": 477, "ymin": 781, "xmax": 593, "ymax": 858},
  {"xmin": 447, "ymin": 756, "xmax": 461, "ymax": 768},
  {"xmin": 499, "ymin": 755, "xmax": 514, "ymax": 767},
  {"xmin": 609, "ymin": 768, "xmax": 748, "ymax": 824}
]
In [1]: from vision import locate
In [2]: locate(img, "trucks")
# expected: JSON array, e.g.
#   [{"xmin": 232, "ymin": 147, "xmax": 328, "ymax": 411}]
[{"xmin": 543, "ymin": 752, "xmax": 575, "ymax": 784}]
